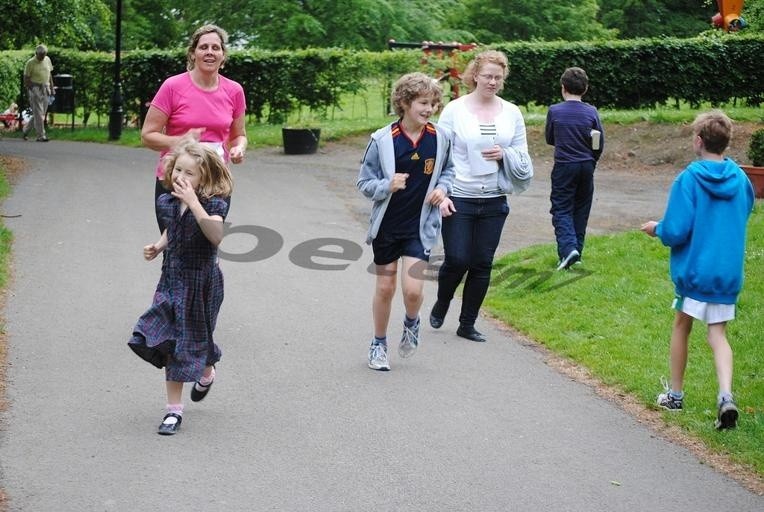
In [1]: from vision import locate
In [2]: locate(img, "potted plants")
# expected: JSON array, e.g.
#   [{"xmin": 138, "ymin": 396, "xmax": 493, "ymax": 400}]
[{"xmin": 734, "ymin": 128, "xmax": 764, "ymax": 198}]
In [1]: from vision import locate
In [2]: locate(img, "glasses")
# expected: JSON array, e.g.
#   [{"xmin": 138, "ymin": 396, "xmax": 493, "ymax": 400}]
[{"xmin": 477, "ymin": 73, "xmax": 504, "ymax": 83}]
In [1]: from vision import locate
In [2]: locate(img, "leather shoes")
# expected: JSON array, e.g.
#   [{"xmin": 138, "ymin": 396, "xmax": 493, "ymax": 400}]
[
  {"xmin": 190, "ymin": 377, "xmax": 213, "ymax": 402},
  {"xmin": 158, "ymin": 412, "xmax": 182, "ymax": 435}
]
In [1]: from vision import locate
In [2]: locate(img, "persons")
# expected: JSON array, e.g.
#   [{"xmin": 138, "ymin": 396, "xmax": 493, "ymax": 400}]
[
  {"xmin": 544, "ymin": 66, "xmax": 604, "ymax": 271},
  {"xmin": 641, "ymin": 109, "xmax": 755, "ymax": 431},
  {"xmin": 1, "ymin": 103, "xmax": 48, "ymax": 133},
  {"xmin": 22, "ymin": 44, "xmax": 56, "ymax": 142},
  {"xmin": 357, "ymin": 71, "xmax": 457, "ymax": 371},
  {"xmin": 428, "ymin": 49, "xmax": 536, "ymax": 344},
  {"xmin": 126, "ymin": 142, "xmax": 235, "ymax": 436},
  {"xmin": 140, "ymin": 23, "xmax": 249, "ymax": 271}
]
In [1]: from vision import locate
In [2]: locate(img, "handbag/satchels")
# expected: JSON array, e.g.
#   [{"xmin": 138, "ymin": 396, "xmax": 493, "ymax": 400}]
[{"xmin": 590, "ymin": 128, "xmax": 601, "ymax": 150}]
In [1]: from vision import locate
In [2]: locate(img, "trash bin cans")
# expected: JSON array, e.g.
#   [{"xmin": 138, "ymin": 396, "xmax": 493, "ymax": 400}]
[{"xmin": 49, "ymin": 73, "xmax": 75, "ymax": 114}]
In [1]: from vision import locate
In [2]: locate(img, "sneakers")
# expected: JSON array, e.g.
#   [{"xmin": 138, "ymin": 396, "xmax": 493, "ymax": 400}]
[
  {"xmin": 36, "ymin": 136, "xmax": 50, "ymax": 143},
  {"xmin": 556, "ymin": 249, "xmax": 583, "ymax": 271},
  {"xmin": 430, "ymin": 303, "xmax": 447, "ymax": 329},
  {"xmin": 656, "ymin": 391, "xmax": 685, "ymax": 412},
  {"xmin": 713, "ymin": 396, "xmax": 739, "ymax": 431},
  {"xmin": 367, "ymin": 340, "xmax": 391, "ymax": 372},
  {"xmin": 456, "ymin": 326, "xmax": 487, "ymax": 343},
  {"xmin": 398, "ymin": 317, "xmax": 420, "ymax": 359},
  {"xmin": 23, "ymin": 126, "xmax": 29, "ymax": 141}
]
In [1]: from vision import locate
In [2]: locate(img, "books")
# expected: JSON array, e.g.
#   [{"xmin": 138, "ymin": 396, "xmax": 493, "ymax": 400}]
[{"xmin": 589, "ymin": 129, "xmax": 602, "ymax": 151}]
